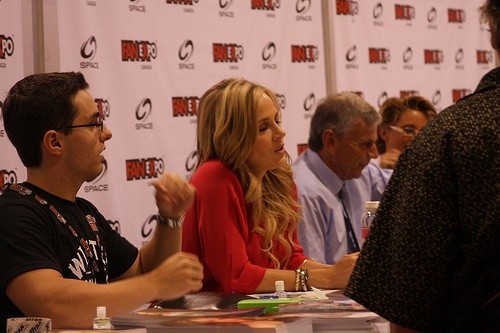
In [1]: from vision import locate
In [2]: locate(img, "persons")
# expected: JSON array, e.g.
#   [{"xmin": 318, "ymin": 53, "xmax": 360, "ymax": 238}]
[
  {"xmin": 343, "ymin": 0, "xmax": 500, "ymax": 333},
  {"xmin": 290, "ymin": 92, "xmax": 380, "ymax": 264},
  {"xmin": 0, "ymin": 72, "xmax": 206, "ymax": 333},
  {"xmin": 358, "ymin": 96, "xmax": 438, "ymax": 203},
  {"xmin": 182, "ymin": 78, "xmax": 360, "ymax": 294}
]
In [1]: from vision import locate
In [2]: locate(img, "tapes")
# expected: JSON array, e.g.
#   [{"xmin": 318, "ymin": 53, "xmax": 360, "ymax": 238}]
[{"xmin": 6, "ymin": 317, "xmax": 52, "ymax": 333}]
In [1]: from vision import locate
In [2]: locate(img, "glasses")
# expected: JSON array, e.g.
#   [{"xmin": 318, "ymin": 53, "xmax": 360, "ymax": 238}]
[
  {"xmin": 41, "ymin": 117, "xmax": 104, "ymax": 144},
  {"xmin": 384, "ymin": 122, "xmax": 423, "ymax": 137}
]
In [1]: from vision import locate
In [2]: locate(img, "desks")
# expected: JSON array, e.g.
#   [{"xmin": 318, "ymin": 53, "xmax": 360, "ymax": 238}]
[{"xmin": 110, "ymin": 309, "xmax": 396, "ymax": 333}]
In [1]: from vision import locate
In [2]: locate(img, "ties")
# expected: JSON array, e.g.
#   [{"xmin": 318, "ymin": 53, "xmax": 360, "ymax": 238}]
[{"xmin": 339, "ymin": 189, "xmax": 359, "ymax": 253}]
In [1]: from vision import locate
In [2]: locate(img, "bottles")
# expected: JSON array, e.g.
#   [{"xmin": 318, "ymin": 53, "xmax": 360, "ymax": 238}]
[
  {"xmin": 93, "ymin": 305, "xmax": 111, "ymax": 330},
  {"xmin": 361, "ymin": 201, "xmax": 382, "ymax": 243},
  {"xmin": 274, "ymin": 280, "xmax": 287, "ymax": 298}
]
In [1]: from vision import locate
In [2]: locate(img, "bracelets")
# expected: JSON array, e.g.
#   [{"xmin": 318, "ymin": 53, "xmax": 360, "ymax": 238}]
[
  {"xmin": 158, "ymin": 213, "xmax": 183, "ymax": 228},
  {"xmin": 298, "ymin": 259, "xmax": 314, "ymax": 269},
  {"xmin": 295, "ymin": 269, "xmax": 312, "ymax": 291}
]
